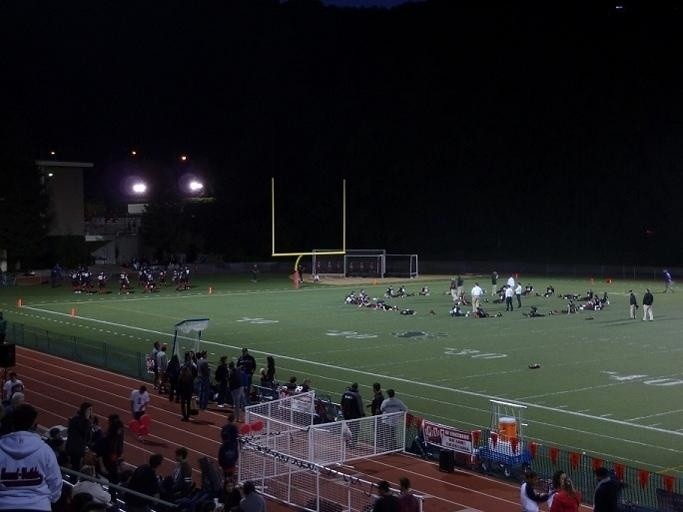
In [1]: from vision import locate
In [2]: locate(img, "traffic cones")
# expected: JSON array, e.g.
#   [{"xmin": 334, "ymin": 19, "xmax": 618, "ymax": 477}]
[
  {"xmin": 207, "ymin": 286, "xmax": 213, "ymax": 295},
  {"xmin": 70, "ymin": 308, "xmax": 77, "ymax": 317},
  {"xmin": 17, "ymin": 298, "xmax": 23, "ymax": 308}
]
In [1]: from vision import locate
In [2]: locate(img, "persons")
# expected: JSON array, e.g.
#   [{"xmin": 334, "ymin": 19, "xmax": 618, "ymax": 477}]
[
  {"xmin": 519, "ymin": 470, "xmax": 557, "ymax": 512},
  {"xmin": 345, "ymin": 271, "xmax": 610, "ymax": 320},
  {"xmin": 544, "ymin": 471, "xmax": 563, "ymax": 511},
  {"xmin": 663, "ymin": 269, "xmax": 674, "ymax": 294},
  {"xmin": 549, "ymin": 470, "xmax": 582, "ymax": 512},
  {"xmin": 642, "ymin": 288, "xmax": 654, "ymax": 320},
  {"xmin": 249, "ymin": 264, "xmax": 259, "ymax": 283},
  {"xmin": 312, "ymin": 259, "xmax": 342, "ymax": 284},
  {"xmin": 591, "ymin": 467, "xmax": 617, "ymax": 512},
  {"xmin": 628, "ymin": 289, "xmax": 637, "ymax": 318},
  {"xmin": 0, "ymin": 337, "xmax": 428, "ymax": 511},
  {"xmin": 297, "ymin": 264, "xmax": 305, "ymax": 283},
  {"xmin": 52, "ymin": 259, "xmax": 193, "ymax": 296}
]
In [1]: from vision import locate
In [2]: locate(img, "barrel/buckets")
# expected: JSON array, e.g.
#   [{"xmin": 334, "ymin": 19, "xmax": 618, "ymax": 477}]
[{"xmin": 498, "ymin": 417, "xmax": 517, "ymax": 438}]
